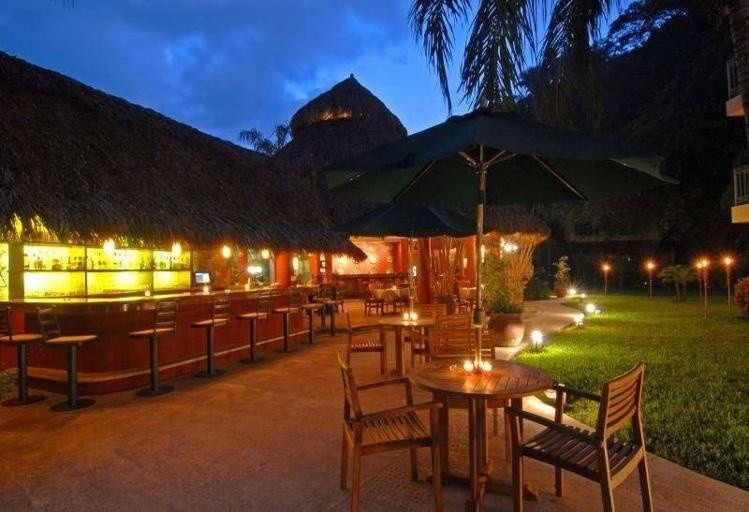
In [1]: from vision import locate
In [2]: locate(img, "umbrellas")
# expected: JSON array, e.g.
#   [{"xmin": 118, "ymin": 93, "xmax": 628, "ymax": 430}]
[
  {"xmin": 332, "ymin": 203, "xmax": 494, "ymax": 318},
  {"xmin": 317, "ymin": 96, "xmax": 681, "ymax": 374}
]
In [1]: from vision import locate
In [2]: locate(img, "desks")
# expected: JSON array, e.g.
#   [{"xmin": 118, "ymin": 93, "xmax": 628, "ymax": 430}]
[
  {"xmin": 377, "ymin": 315, "xmax": 435, "ymax": 377},
  {"xmin": 409, "ymin": 356, "xmax": 555, "ymax": 511}
]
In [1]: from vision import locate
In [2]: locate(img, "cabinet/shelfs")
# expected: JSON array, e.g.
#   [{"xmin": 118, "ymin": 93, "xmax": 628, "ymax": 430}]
[{"xmin": 0, "ymin": 237, "xmax": 197, "ymax": 296}]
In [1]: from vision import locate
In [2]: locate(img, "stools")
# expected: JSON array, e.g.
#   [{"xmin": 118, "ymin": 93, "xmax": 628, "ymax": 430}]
[
  {"xmin": 129, "ymin": 297, "xmax": 182, "ymax": 397},
  {"xmin": 37, "ymin": 304, "xmax": 98, "ymax": 410},
  {"xmin": 235, "ymin": 288, "xmax": 272, "ymax": 366},
  {"xmin": 300, "ymin": 289, "xmax": 325, "ymax": 345},
  {"xmin": 1, "ymin": 306, "xmax": 50, "ymax": 406},
  {"xmin": 272, "ymin": 290, "xmax": 301, "ymax": 353},
  {"xmin": 190, "ymin": 292, "xmax": 234, "ymax": 380}
]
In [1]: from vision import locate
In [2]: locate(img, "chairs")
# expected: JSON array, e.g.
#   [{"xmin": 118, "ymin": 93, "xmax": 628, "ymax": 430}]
[
  {"xmin": 414, "ymin": 312, "xmax": 471, "ymax": 362},
  {"xmin": 344, "ymin": 312, "xmax": 387, "ymax": 381},
  {"xmin": 335, "ymin": 347, "xmax": 440, "ymax": 505},
  {"xmin": 429, "ymin": 327, "xmax": 503, "ymax": 435},
  {"xmin": 311, "ymin": 271, "xmax": 491, "ymax": 334},
  {"xmin": 505, "ymin": 361, "xmax": 652, "ymax": 510}
]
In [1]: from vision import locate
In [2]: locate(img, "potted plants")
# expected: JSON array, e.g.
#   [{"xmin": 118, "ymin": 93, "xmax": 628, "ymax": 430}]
[
  {"xmin": 552, "ymin": 255, "xmax": 571, "ymax": 298},
  {"xmin": 482, "ymin": 231, "xmax": 525, "ymax": 346}
]
